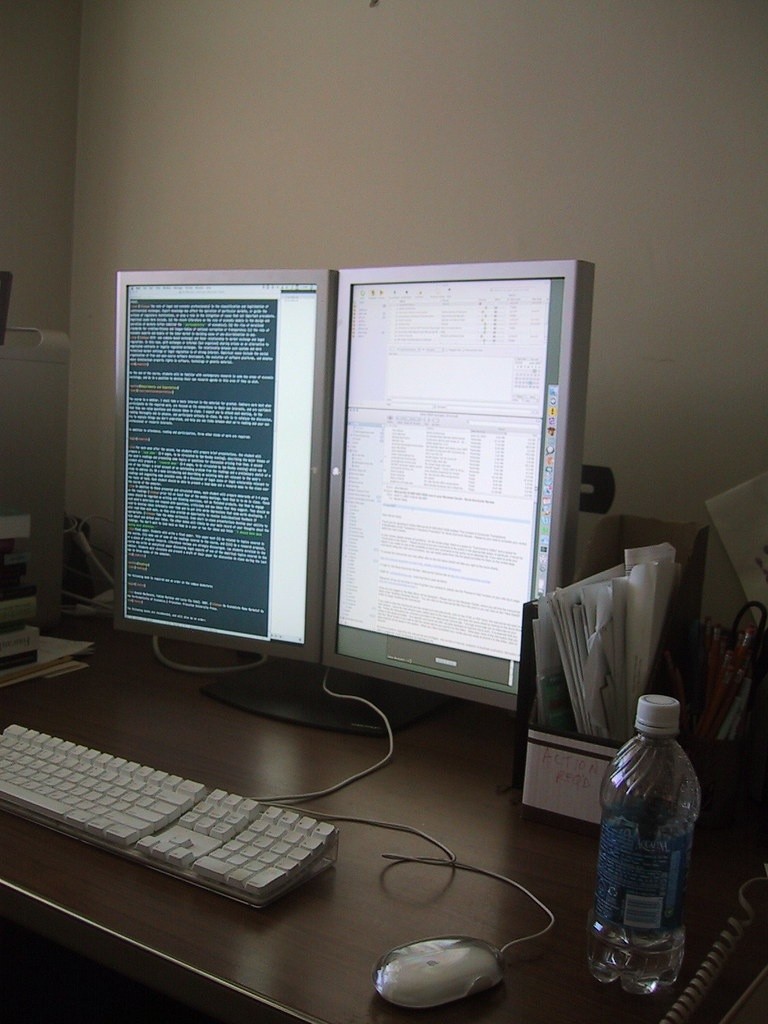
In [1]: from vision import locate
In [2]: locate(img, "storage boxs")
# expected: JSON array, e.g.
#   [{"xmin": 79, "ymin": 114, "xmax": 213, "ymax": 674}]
[{"xmin": 521, "ymin": 515, "xmax": 709, "ymax": 825}]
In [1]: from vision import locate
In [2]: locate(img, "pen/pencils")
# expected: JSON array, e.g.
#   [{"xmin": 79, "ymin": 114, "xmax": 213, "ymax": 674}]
[{"xmin": 665, "ymin": 613, "xmax": 758, "ymax": 740}]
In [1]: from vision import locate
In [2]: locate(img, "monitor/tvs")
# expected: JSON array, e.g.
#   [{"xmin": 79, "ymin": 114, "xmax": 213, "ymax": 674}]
[{"xmin": 108, "ymin": 259, "xmax": 596, "ymax": 736}]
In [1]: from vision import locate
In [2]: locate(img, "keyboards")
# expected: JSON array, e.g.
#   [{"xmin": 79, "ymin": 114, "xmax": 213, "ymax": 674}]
[{"xmin": 0, "ymin": 723, "xmax": 341, "ymax": 909}]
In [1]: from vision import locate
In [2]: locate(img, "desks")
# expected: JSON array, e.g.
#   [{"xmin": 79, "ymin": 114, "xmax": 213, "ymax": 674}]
[{"xmin": 0, "ymin": 605, "xmax": 768, "ymax": 1024}]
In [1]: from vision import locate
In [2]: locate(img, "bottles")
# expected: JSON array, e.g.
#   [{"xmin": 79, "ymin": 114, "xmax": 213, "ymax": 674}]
[{"xmin": 585, "ymin": 693, "xmax": 701, "ymax": 996}]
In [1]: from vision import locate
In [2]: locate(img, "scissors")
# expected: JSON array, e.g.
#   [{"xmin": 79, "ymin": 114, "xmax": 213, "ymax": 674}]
[{"xmin": 728, "ymin": 600, "xmax": 768, "ymax": 711}]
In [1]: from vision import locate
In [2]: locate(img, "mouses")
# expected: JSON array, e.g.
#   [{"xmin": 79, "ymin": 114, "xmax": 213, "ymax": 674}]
[{"xmin": 371, "ymin": 934, "xmax": 505, "ymax": 1009}]
